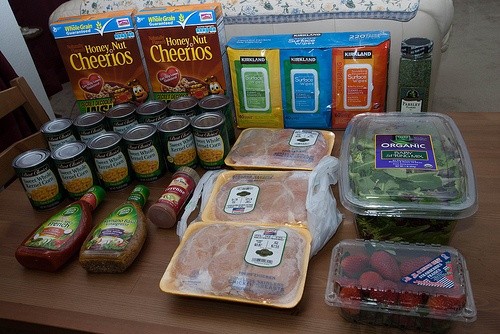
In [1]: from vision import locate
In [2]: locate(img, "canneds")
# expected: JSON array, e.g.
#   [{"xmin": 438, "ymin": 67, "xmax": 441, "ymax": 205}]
[
  {"xmin": 87, "ymin": 131, "xmax": 133, "ymax": 192},
  {"xmin": 191, "ymin": 112, "xmax": 230, "ymax": 169},
  {"xmin": 137, "ymin": 101, "xmax": 167, "ymax": 124},
  {"xmin": 13, "ymin": 148, "xmax": 66, "ymax": 212},
  {"xmin": 105, "ymin": 104, "xmax": 137, "ymax": 131},
  {"xmin": 167, "ymin": 97, "xmax": 198, "ymax": 117},
  {"xmin": 40, "ymin": 119, "xmax": 75, "ymax": 151},
  {"xmin": 157, "ymin": 115, "xmax": 198, "ymax": 171},
  {"xmin": 51, "ymin": 142, "xmax": 97, "ymax": 200},
  {"xmin": 123, "ymin": 124, "xmax": 164, "ymax": 183},
  {"xmin": 199, "ymin": 94, "xmax": 234, "ymax": 143},
  {"xmin": 73, "ymin": 112, "xmax": 106, "ymax": 143}
]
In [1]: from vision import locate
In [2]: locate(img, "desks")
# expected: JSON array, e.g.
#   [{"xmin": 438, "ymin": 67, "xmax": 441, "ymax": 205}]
[{"xmin": 0, "ymin": 110, "xmax": 500, "ymax": 334}]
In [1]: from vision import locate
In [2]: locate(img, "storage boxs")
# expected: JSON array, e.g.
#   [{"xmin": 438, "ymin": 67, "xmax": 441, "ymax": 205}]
[
  {"xmin": 135, "ymin": 3, "xmax": 235, "ymax": 126},
  {"xmin": 48, "ymin": 11, "xmax": 150, "ymax": 117}
]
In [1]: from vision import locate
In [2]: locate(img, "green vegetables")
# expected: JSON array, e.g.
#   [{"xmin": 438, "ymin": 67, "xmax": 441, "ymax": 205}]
[{"xmin": 349, "ymin": 122, "xmax": 461, "ymax": 245}]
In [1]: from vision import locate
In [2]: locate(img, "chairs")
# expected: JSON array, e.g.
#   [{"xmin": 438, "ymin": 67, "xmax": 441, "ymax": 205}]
[{"xmin": 0, "ymin": 77, "xmax": 51, "ymax": 190}]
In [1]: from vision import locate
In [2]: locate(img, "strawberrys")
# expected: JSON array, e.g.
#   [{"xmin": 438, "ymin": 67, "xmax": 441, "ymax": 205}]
[{"xmin": 339, "ymin": 251, "xmax": 466, "ymax": 315}]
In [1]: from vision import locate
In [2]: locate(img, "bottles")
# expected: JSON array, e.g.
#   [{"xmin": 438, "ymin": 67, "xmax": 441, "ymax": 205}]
[
  {"xmin": 15, "ymin": 186, "xmax": 104, "ymax": 272},
  {"xmin": 78, "ymin": 184, "xmax": 152, "ymax": 274},
  {"xmin": 150, "ymin": 165, "xmax": 200, "ymax": 230}
]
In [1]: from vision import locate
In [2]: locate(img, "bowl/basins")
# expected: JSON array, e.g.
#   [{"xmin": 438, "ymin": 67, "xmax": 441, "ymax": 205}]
[
  {"xmin": 339, "ymin": 109, "xmax": 480, "ymax": 247},
  {"xmin": 325, "ymin": 239, "xmax": 477, "ymax": 333}
]
